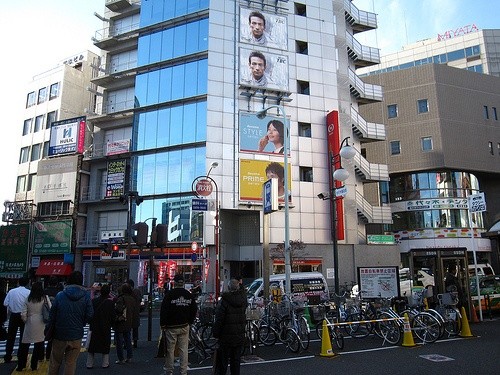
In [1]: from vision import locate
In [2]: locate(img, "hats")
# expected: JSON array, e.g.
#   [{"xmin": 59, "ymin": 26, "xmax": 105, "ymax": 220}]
[
  {"xmin": 228, "ymin": 278, "xmax": 240, "ymax": 291},
  {"xmin": 174, "ymin": 274, "xmax": 183, "ymax": 282}
]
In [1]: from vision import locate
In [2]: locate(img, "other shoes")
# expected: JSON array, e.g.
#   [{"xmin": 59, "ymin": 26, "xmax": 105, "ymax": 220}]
[
  {"xmin": 16, "ymin": 362, "xmax": 26, "ymax": 371},
  {"xmin": 116, "ymin": 359, "xmax": 125, "ymax": 364},
  {"xmin": 125, "ymin": 358, "xmax": 132, "ymax": 362}
]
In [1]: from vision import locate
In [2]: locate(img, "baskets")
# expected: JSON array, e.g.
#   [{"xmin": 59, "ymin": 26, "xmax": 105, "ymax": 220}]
[
  {"xmin": 274, "ymin": 301, "xmax": 290, "ymax": 319},
  {"xmin": 346, "ymin": 299, "xmax": 360, "ymax": 314},
  {"xmin": 245, "ymin": 305, "xmax": 260, "ymax": 320},
  {"xmin": 309, "ymin": 305, "xmax": 324, "ymax": 322},
  {"xmin": 198, "ymin": 306, "xmax": 214, "ymax": 323}
]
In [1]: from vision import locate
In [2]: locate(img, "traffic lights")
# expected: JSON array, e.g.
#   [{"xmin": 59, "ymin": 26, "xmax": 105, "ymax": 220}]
[
  {"xmin": 113, "ymin": 245, "xmax": 119, "ymax": 257},
  {"xmin": 130, "ymin": 221, "xmax": 148, "ymax": 247}
]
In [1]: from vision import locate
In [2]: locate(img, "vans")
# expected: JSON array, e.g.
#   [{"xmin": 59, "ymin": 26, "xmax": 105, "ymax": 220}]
[
  {"xmin": 468, "ymin": 263, "xmax": 496, "ymax": 278},
  {"xmin": 245, "ymin": 271, "xmax": 331, "ymax": 319}
]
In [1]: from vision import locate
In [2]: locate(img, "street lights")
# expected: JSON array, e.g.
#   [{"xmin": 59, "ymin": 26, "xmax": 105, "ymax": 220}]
[
  {"xmin": 256, "ymin": 104, "xmax": 292, "ymax": 295},
  {"xmin": 317, "ymin": 135, "xmax": 356, "ymax": 320}
]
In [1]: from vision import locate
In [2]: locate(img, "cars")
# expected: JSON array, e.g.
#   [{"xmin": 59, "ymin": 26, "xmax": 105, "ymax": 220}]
[
  {"xmin": 349, "ymin": 268, "xmax": 435, "ymax": 301},
  {"xmin": 469, "ymin": 274, "xmax": 500, "ymax": 312},
  {"xmin": 85, "ymin": 288, "xmax": 116, "ymax": 300}
]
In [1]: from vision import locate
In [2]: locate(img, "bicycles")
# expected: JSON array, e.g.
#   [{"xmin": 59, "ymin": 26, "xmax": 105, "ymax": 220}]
[{"xmin": 187, "ymin": 280, "xmax": 464, "ymax": 367}]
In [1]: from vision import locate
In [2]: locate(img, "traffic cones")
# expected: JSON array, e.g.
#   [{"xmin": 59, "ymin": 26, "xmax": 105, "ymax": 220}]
[
  {"xmin": 319, "ymin": 319, "xmax": 334, "ymax": 357},
  {"xmin": 458, "ymin": 306, "xmax": 473, "ymax": 337},
  {"xmin": 471, "ymin": 305, "xmax": 480, "ymax": 324},
  {"xmin": 401, "ymin": 313, "xmax": 416, "ymax": 347}
]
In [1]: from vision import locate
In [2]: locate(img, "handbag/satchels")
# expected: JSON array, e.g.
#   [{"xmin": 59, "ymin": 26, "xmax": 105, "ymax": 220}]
[
  {"xmin": 84, "ymin": 329, "xmax": 93, "ymax": 348},
  {"xmin": 43, "ymin": 296, "xmax": 50, "ymax": 323},
  {"xmin": 114, "ymin": 296, "xmax": 127, "ymax": 322}
]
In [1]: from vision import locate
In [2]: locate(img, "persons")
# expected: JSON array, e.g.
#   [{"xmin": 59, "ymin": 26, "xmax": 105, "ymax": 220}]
[
  {"xmin": 240, "ymin": 11, "xmax": 291, "ymax": 199},
  {"xmin": 160, "ymin": 275, "xmax": 197, "ymax": 375},
  {"xmin": 212, "ymin": 279, "xmax": 247, "ymax": 375},
  {"xmin": 45, "ymin": 272, "xmax": 94, "ymax": 375},
  {"xmin": 16, "ymin": 283, "xmax": 51, "ymax": 371},
  {"xmin": 0, "ymin": 276, "xmax": 142, "ymax": 369}
]
